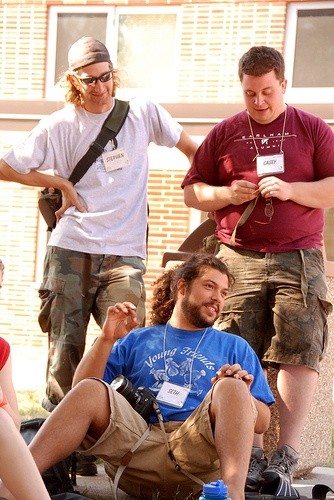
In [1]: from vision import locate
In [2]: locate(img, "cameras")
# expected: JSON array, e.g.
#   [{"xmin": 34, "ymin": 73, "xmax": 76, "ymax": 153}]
[{"xmin": 109, "ymin": 374, "xmax": 157, "ymax": 420}]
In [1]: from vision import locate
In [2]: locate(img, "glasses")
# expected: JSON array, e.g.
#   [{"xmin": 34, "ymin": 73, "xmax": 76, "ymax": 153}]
[{"xmin": 79, "ymin": 69, "xmax": 112, "ymax": 86}]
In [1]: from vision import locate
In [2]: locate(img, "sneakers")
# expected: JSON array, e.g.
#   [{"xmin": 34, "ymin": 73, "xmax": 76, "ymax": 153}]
[
  {"xmin": 245, "ymin": 445, "xmax": 268, "ymax": 490},
  {"xmin": 261, "ymin": 443, "xmax": 300, "ymax": 487}
]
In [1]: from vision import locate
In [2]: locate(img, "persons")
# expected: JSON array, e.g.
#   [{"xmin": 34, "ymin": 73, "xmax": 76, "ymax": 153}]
[
  {"xmin": 0, "ymin": 36, "xmax": 201, "ymax": 415},
  {"xmin": 179, "ymin": 46, "xmax": 334, "ymax": 487},
  {"xmin": 0, "ymin": 254, "xmax": 275, "ymax": 500},
  {"xmin": 0, "ymin": 261, "xmax": 51, "ymax": 500}
]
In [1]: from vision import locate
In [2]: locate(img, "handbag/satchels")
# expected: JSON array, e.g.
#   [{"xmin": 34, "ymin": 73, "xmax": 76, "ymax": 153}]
[{"xmin": 38, "ymin": 186, "xmax": 62, "ymax": 232}]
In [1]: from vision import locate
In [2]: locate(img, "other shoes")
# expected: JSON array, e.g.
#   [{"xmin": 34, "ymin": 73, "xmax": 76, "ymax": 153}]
[{"xmin": 68, "ymin": 460, "xmax": 98, "ymax": 476}]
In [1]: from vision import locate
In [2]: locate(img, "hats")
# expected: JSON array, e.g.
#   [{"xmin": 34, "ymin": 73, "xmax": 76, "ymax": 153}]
[{"xmin": 68, "ymin": 36, "xmax": 111, "ymax": 72}]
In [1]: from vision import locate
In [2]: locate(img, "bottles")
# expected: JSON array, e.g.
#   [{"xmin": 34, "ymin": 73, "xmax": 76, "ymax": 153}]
[{"xmin": 199, "ymin": 479, "xmax": 232, "ymax": 500}]
